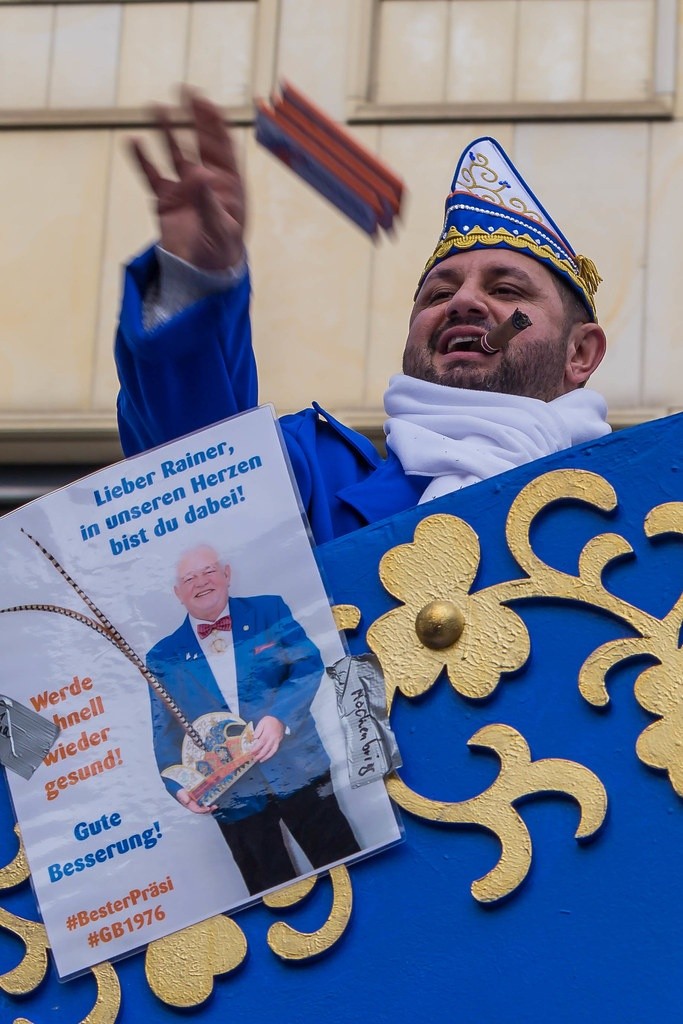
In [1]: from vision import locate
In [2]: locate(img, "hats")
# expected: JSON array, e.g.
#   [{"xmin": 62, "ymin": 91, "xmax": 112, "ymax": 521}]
[{"xmin": 412, "ymin": 132, "xmax": 600, "ymax": 324}]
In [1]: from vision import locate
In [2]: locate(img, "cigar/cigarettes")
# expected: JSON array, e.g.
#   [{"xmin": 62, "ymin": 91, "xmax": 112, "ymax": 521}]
[{"xmin": 462, "ymin": 309, "xmax": 532, "ymax": 355}]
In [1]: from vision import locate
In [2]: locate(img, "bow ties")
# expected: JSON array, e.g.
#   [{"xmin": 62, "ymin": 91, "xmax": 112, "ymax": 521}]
[{"xmin": 196, "ymin": 615, "xmax": 232, "ymax": 641}]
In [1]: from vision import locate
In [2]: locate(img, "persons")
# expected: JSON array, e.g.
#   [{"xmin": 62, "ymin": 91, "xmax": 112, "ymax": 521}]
[
  {"xmin": 145, "ymin": 545, "xmax": 363, "ymax": 894},
  {"xmin": 112, "ymin": 89, "xmax": 610, "ymax": 551}
]
